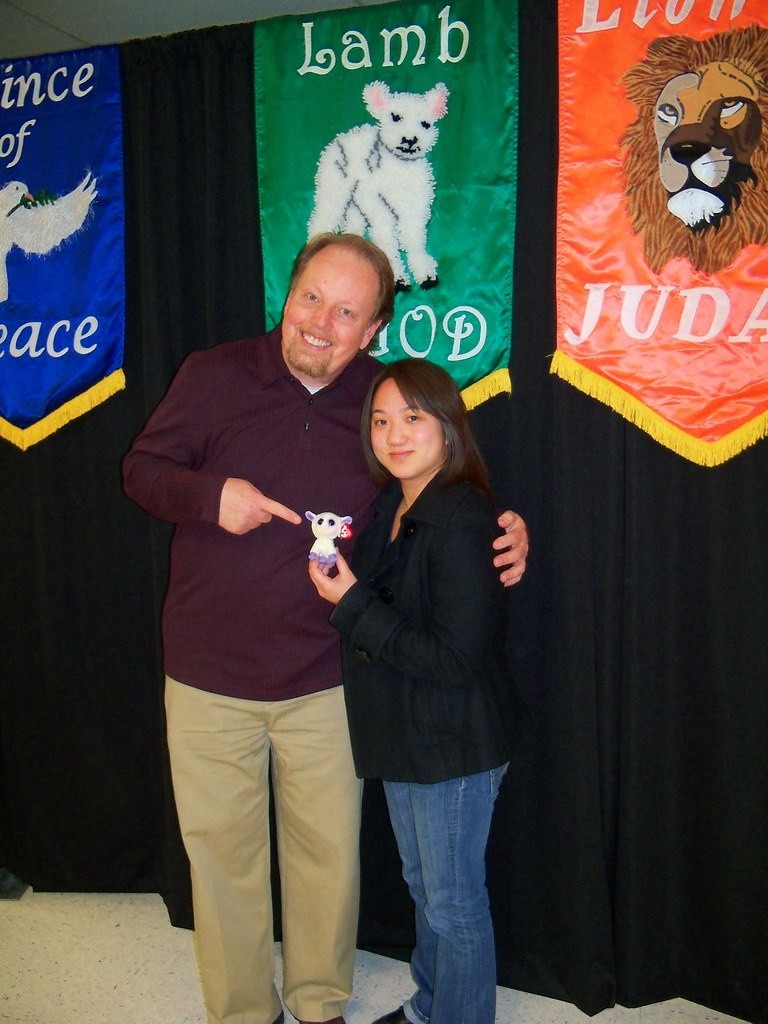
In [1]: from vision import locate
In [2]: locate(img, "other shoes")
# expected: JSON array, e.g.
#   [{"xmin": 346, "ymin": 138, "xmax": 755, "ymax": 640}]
[
  {"xmin": 372, "ymin": 1005, "xmax": 414, "ymax": 1024},
  {"xmin": 299, "ymin": 1015, "xmax": 345, "ymax": 1024},
  {"xmin": 272, "ymin": 1009, "xmax": 285, "ymax": 1024}
]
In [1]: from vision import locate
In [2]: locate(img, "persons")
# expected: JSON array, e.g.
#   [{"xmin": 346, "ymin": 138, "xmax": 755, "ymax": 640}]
[
  {"xmin": 124, "ymin": 234, "xmax": 532, "ymax": 1024},
  {"xmin": 310, "ymin": 358, "xmax": 514, "ymax": 1023}
]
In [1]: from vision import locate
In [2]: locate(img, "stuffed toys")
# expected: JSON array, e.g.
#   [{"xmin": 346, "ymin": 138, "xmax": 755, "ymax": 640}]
[{"xmin": 305, "ymin": 511, "xmax": 352, "ymax": 565}]
